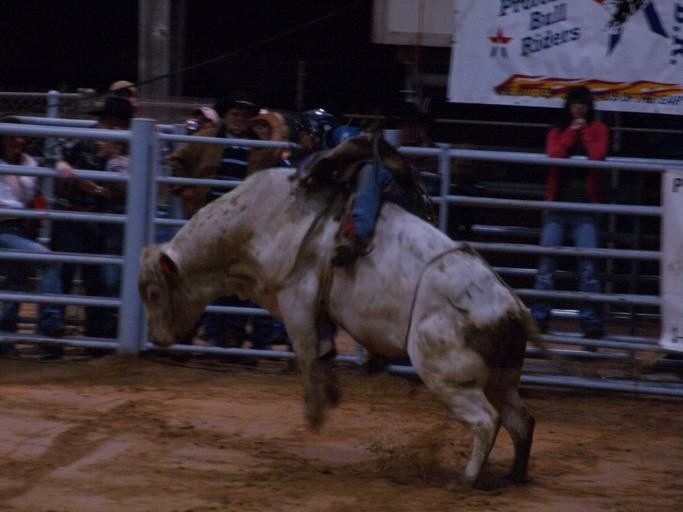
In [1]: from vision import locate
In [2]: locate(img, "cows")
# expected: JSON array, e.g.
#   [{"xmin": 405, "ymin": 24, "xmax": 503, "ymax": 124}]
[{"xmin": 135, "ymin": 165, "xmax": 553, "ymax": 492}]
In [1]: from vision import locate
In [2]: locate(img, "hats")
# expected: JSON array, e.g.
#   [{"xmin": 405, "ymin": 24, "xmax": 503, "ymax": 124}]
[
  {"xmin": 189, "ymin": 95, "xmax": 260, "ymax": 124},
  {"xmin": 87, "ymin": 81, "xmax": 139, "ymax": 117}
]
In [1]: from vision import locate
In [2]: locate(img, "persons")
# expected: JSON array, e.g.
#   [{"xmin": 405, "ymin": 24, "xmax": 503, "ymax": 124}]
[
  {"xmin": 158, "ymin": 92, "xmax": 459, "ymax": 373},
  {"xmin": 1, "ymin": 79, "xmax": 141, "ymax": 359},
  {"xmin": 519, "ymin": 86, "xmax": 614, "ymax": 339}
]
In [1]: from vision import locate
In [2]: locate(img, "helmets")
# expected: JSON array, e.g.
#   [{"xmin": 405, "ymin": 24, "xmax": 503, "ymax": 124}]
[{"xmin": 296, "ymin": 108, "xmax": 336, "ymax": 137}]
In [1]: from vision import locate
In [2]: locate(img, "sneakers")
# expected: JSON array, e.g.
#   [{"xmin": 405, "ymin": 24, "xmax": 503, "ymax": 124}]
[{"xmin": 333, "ymin": 244, "xmax": 351, "ymax": 268}]
